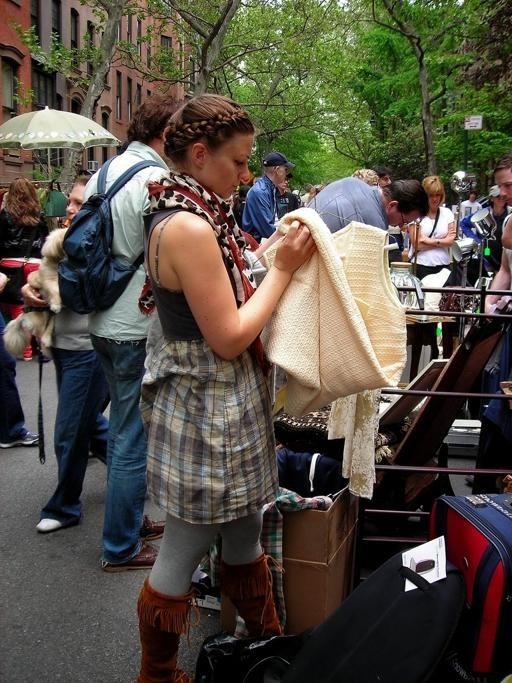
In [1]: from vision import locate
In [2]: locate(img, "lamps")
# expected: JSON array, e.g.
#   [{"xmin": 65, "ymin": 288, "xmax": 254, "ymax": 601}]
[
  {"xmin": 450, "ymin": 170, "xmax": 473, "ymax": 240},
  {"xmin": 450, "ymin": 235, "xmax": 479, "ymax": 342},
  {"xmin": 470, "ymin": 206, "xmax": 499, "ymax": 289}
]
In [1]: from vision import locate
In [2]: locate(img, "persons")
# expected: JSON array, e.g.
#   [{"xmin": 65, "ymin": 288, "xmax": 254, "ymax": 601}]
[
  {"xmin": 78, "ymin": 92, "xmax": 186, "ymax": 576},
  {"xmin": 233, "ymin": 148, "xmax": 512, "ymax": 492},
  {"xmin": 0, "ymin": 169, "xmax": 110, "ymax": 537},
  {"xmin": 137, "ymin": 93, "xmax": 316, "ymax": 682}
]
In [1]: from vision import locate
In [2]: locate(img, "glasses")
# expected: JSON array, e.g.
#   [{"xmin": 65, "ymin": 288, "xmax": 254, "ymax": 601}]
[{"xmin": 398, "ymin": 211, "xmax": 407, "ymax": 228}]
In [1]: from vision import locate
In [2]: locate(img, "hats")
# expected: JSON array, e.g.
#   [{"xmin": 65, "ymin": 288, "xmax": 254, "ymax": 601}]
[
  {"xmin": 489, "ymin": 185, "xmax": 501, "ymax": 197},
  {"xmin": 262, "ymin": 152, "xmax": 296, "ymax": 169}
]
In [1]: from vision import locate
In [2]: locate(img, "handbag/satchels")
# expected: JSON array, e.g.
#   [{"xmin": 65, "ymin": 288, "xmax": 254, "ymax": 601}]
[
  {"xmin": 282, "ymin": 548, "xmax": 463, "ymax": 681},
  {"xmin": 40, "ymin": 179, "xmax": 67, "ymax": 216},
  {"xmin": 0, "ymin": 266, "xmax": 25, "ymax": 305}
]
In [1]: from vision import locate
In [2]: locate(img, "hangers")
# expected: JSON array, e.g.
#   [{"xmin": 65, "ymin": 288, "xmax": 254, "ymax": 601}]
[{"xmin": 251, "ymin": 182, "xmax": 398, "ymax": 270}]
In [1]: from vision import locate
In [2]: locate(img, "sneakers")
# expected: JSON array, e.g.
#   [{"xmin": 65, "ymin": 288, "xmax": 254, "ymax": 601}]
[{"xmin": 0, "ymin": 433, "xmax": 39, "ymax": 447}]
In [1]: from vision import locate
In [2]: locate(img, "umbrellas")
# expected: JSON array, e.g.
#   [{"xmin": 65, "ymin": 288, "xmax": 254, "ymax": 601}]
[{"xmin": 0, "ymin": 105, "xmax": 121, "ymax": 179}]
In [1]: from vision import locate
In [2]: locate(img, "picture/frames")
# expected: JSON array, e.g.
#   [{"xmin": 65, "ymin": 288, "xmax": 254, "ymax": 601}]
[{"xmin": 378, "ymin": 357, "xmax": 448, "ymax": 432}]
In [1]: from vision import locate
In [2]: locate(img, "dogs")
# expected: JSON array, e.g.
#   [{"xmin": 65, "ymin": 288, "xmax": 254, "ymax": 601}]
[{"xmin": 2, "ymin": 227, "xmax": 70, "ymax": 361}]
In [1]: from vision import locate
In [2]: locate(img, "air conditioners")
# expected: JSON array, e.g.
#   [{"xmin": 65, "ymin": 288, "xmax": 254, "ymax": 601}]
[{"xmin": 88, "ymin": 160, "xmax": 99, "ymax": 171}]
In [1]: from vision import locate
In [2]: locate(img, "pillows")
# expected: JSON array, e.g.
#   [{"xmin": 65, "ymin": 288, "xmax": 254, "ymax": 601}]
[{"xmin": 269, "ymin": 396, "xmax": 411, "ymax": 465}]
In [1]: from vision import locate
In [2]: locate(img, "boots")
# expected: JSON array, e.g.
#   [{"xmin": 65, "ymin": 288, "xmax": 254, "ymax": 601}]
[
  {"xmin": 137, "ymin": 578, "xmax": 195, "ymax": 682},
  {"xmin": 215, "ymin": 552, "xmax": 283, "ymax": 638}
]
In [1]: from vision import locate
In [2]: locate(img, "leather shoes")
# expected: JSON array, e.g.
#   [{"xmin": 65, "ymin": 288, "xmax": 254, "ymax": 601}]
[
  {"xmin": 34, "ymin": 519, "xmax": 62, "ymax": 531},
  {"xmin": 140, "ymin": 516, "xmax": 166, "ymax": 540},
  {"xmin": 101, "ymin": 538, "xmax": 160, "ymax": 572}
]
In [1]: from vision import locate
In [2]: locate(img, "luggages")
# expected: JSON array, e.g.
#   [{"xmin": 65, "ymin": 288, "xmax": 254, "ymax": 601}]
[{"xmin": 429, "ymin": 493, "xmax": 512, "ymax": 683}]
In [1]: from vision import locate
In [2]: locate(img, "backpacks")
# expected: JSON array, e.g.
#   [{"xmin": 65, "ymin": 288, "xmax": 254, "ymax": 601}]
[{"xmin": 58, "ymin": 154, "xmax": 163, "ymax": 314}]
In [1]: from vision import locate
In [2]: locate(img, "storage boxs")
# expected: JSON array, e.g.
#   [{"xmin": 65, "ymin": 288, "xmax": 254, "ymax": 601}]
[{"xmin": 280, "ymin": 481, "xmax": 359, "ymax": 636}]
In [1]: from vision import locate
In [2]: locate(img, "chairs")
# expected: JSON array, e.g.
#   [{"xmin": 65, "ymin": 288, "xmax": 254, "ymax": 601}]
[{"xmin": 362, "ymin": 295, "xmax": 512, "ymax": 531}]
